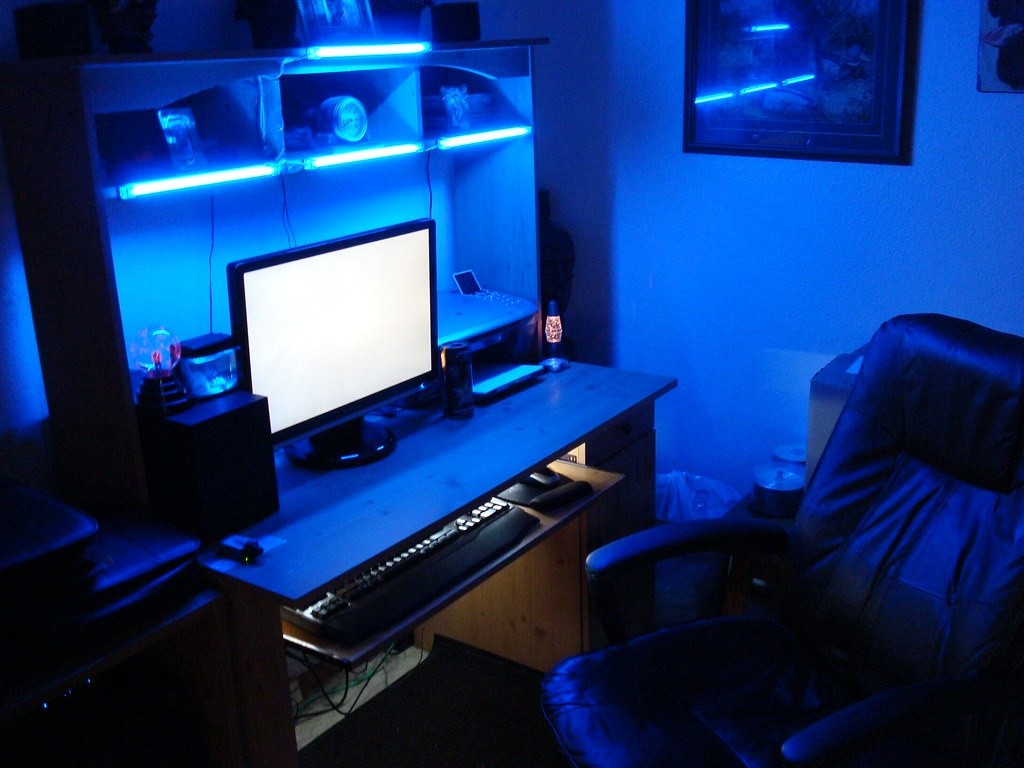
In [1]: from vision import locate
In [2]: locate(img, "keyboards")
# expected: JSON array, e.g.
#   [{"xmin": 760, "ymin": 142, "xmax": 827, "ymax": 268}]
[{"xmin": 280, "ymin": 499, "xmax": 540, "ymax": 645}]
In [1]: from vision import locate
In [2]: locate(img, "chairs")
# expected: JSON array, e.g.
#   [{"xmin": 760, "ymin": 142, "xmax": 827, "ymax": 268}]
[{"xmin": 538, "ymin": 314, "xmax": 1024, "ymax": 768}]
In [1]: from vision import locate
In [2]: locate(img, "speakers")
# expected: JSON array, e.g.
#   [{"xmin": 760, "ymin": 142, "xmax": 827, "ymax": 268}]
[{"xmin": 138, "ymin": 386, "xmax": 279, "ymax": 552}]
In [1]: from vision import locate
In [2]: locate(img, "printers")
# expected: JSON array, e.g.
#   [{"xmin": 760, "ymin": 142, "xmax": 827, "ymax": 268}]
[{"xmin": 399, "ymin": 268, "xmax": 545, "ymax": 403}]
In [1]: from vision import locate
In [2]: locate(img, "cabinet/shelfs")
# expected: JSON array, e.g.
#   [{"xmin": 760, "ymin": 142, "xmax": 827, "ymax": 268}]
[{"xmin": 77, "ymin": 37, "xmax": 550, "ymax": 412}]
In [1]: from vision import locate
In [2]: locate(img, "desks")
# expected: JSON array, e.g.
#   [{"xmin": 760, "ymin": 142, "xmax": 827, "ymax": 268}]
[{"xmin": 196, "ymin": 358, "xmax": 678, "ymax": 768}]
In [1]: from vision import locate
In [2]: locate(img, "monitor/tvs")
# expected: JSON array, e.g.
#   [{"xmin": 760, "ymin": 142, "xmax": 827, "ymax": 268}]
[{"xmin": 227, "ymin": 217, "xmax": 440, "ymax": 470}]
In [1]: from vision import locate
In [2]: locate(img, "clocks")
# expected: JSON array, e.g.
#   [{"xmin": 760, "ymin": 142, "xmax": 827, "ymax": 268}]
[{"xmin": 318, "ymin": 95, "xmax": 367, "ymax": 144}]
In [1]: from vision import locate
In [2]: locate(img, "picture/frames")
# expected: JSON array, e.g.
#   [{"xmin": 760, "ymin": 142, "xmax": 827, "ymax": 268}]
[
  {"xmin": 156, "ymin": 105, "xmax": 208, "ymax": 173},
  {"xmin": 296, "ymin": 0, "xmax": 378, "ymax": 47},
  {"xmin": 682, "ymin": 0, "xmax": 924, "ymax": 166}
]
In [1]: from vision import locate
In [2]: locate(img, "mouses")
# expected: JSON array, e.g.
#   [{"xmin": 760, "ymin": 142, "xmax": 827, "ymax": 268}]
[{"xmin": 519, "ymin": 467, "xmax": 562, "ymax": 490}]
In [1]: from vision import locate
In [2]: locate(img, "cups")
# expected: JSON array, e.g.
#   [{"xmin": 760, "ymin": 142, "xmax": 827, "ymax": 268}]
[{"xmin": 750, "ymin": 463, "xmax": 806, "ymax": 516}]
